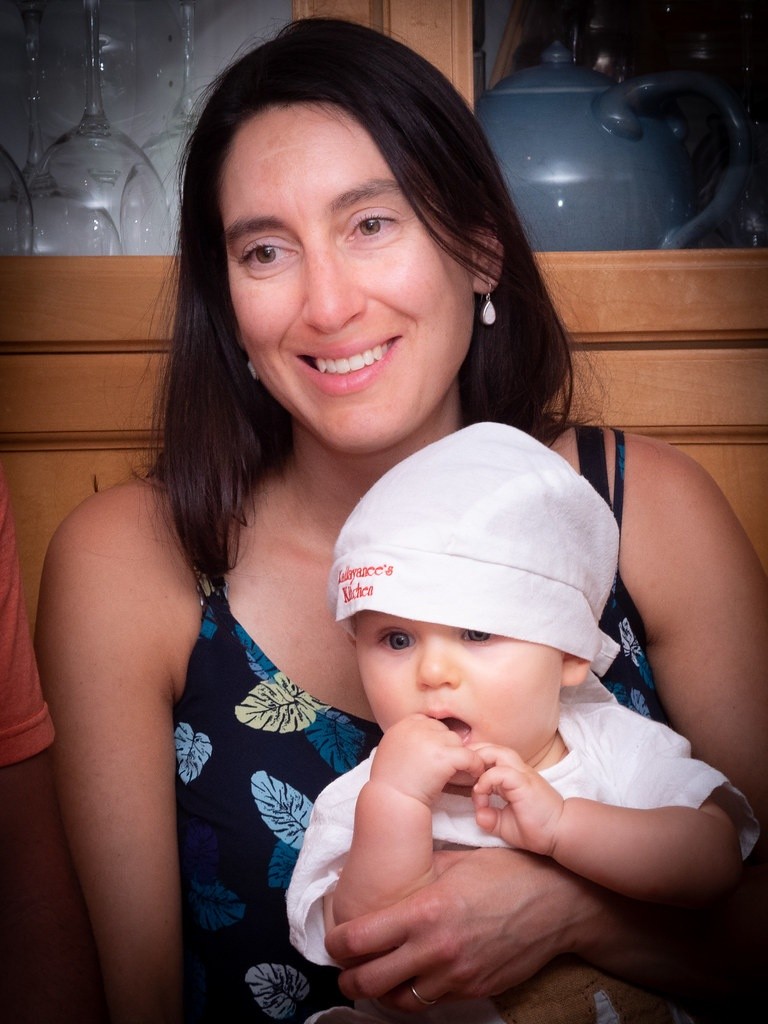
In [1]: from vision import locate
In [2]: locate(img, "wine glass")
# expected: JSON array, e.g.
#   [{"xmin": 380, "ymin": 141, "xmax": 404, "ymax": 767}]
[{"xmin": 0, "ymin": 0, "xmax": 198, "ymax": 257}]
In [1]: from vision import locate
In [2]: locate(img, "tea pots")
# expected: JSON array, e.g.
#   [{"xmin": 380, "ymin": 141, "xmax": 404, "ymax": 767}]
[{"xmin": 476, "ymin": 37, "xmax": 751, "ymax": 250}]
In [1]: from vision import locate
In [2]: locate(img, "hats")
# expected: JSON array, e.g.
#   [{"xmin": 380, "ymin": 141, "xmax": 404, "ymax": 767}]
[{"xmin": 328, "ymin": 422, "xmax": 621, "ymax": 676}]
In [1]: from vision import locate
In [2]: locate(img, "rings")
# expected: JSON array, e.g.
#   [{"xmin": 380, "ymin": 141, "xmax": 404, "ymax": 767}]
[{"xmin": 408, "ymin": 979, "xmax": 438, "ymax": 1004}]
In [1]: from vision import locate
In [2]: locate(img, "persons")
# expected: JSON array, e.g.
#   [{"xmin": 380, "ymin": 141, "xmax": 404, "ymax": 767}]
[
  {"xmin": 0, "ymin": 467, "xmax": 108, "ymax": 1024},
  {"xmin": 285, "ymin": 415, "xmax": 759, "ymax": 969},
  {"xmin": 33, "ymin": 16, "xmax": 767, "ymax": 1024}
]
